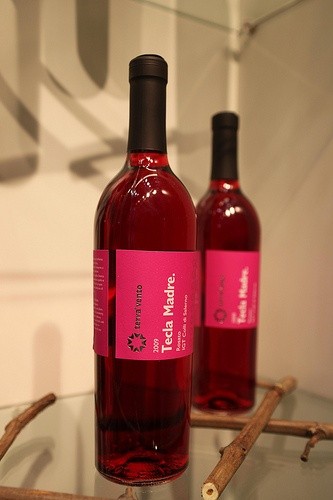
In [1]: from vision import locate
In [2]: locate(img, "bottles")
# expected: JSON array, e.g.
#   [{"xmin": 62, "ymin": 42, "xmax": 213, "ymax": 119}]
[
  {"xmin": 192, "ymin": 109, "xmax": 262, "ymax": 415},
  {"xmin": 92, "ymin": 53, "xmax": 196, "ymax": 488}
]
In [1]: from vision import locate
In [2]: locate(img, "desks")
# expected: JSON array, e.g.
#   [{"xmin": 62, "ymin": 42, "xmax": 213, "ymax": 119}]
[{"xmin": 0, "ymin": 377, "xmax": 333, "ymax": 500}]
